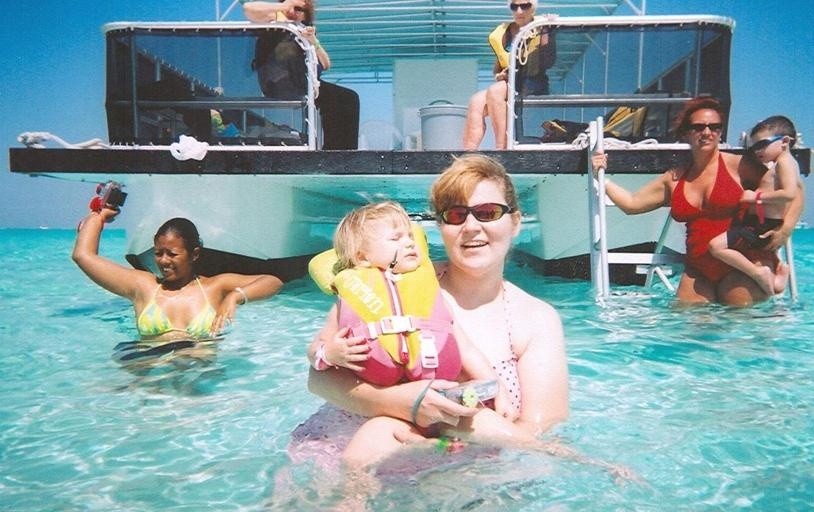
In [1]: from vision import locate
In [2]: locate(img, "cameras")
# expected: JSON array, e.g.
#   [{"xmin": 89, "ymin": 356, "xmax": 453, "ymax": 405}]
[
  {"xmin": 96, "ymin": 181, "xmax": 127, "ymax": 210},
  {"xmin": 439, "ymin": 379, "xmax": 499, "ymax": 408}
]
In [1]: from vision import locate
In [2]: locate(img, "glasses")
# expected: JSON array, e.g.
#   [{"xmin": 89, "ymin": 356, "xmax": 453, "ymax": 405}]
[
  {"xmin": 746, "ymin": 133, "xmax": 795, "ymax": 155},
  {"xmin": 509, "ymin": 3, "xmax": 533, "ymax": 12},
  {"xmin": 293, "ymin": 5, "xmax": 307, "ymax": 14},
  {"xmin": 689, "ymin": 122, "xmax": 723, "ymax": 132},
  {"xmin": 438, "ymin": 202, "xmax": 513, "ymax": 226}
]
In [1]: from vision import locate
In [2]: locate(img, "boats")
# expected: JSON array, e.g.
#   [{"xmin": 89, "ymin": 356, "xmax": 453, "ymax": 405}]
[{"xmin": 7, "ymin": 0, "xmax": 809, "ymax": 295}]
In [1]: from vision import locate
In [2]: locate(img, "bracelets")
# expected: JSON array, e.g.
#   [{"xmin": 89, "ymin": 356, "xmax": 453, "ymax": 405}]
[
  {"xmin": 412, "ymin": 375, "xmax": 434, "ymax": 429},
  {"xmin": 314, "ymin": 340, "xmax": 338, "ymax": 371},
  {"xmin": 234, "ymin": 286, "xmax": 248, "ymax": 305},
  {"xmin": 77, "ymin": 212, "xmax": 104, "ymax": 231},
  {"xmin": 314, "ymin": 39, "xmax": 320, "ymax": 50},
  {"xmin": 756, "ymin": 191, "xmax": 765, "ymax": 224}
]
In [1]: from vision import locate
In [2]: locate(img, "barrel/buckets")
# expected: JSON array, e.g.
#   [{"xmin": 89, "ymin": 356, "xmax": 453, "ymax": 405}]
[{"xmin": 419, "ymin": 100, "xmax": 468, "ymax": 152}]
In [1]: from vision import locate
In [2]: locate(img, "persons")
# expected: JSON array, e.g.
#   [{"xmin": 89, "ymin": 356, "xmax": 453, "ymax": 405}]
[
  {"xmin": 463, "ymin": 0, "xmax": 560, "ymax": 152},
  {"xmin": 109, "ymin": 339, "xmax": 226, "ymax": 396},
  {"xmin": 708, "ymin": 115, "xmax": 801, "ymax": 298},
  {"xmin": 590, "ymin": 95, "xmax": 805, "ymax": 308},
  {"xmin": 271, "ymin": 151, "xmax": 571, "ymax": 512},
  {"xmin": 70, "ymin": 199, "xmax": 285, "ymax": 344},
  {"xmin": 306, "ymin": 201, "xmax": 642, "ymax": 512},
  {"xmin": 243, "ymin": 0, "xmax": 360, "ymax": 151}
]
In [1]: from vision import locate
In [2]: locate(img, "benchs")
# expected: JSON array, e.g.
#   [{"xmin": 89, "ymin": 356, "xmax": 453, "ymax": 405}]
[
  {"xmin": 106, "ymin": 92, "xmax": 309, "ymax": 143},
  {"xmin": 515, "ymin": 91, "xmax": 707, "ymax": 145}
]
